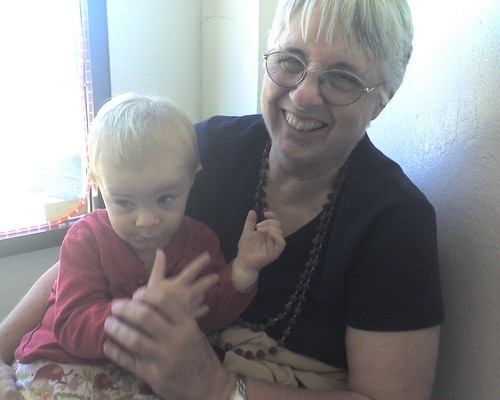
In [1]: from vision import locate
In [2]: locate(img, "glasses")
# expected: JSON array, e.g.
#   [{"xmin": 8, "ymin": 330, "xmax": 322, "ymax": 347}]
[{"xmin": 262, "ymin": 48, "xmax": 386, "ymax": 107}]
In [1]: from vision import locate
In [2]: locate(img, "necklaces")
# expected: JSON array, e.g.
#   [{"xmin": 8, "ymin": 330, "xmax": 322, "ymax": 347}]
[{"xmin": 210, "ymin": 141, "xmax": 352, "ymax": 363}]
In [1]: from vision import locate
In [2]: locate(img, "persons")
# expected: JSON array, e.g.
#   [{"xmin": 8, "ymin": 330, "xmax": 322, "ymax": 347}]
[
  {"xmin": 0, "ymin": 0, "xmax": 446, "ymax": 400},
  {"xmin": 11, "ymin": 91, "xmax": 287, "ymax": 400}
]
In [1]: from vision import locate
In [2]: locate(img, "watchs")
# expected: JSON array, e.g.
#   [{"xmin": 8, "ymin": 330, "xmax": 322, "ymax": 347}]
[{"xmin": 230, "ymin": 373, "xmax": 247, "ymax": 400}]
